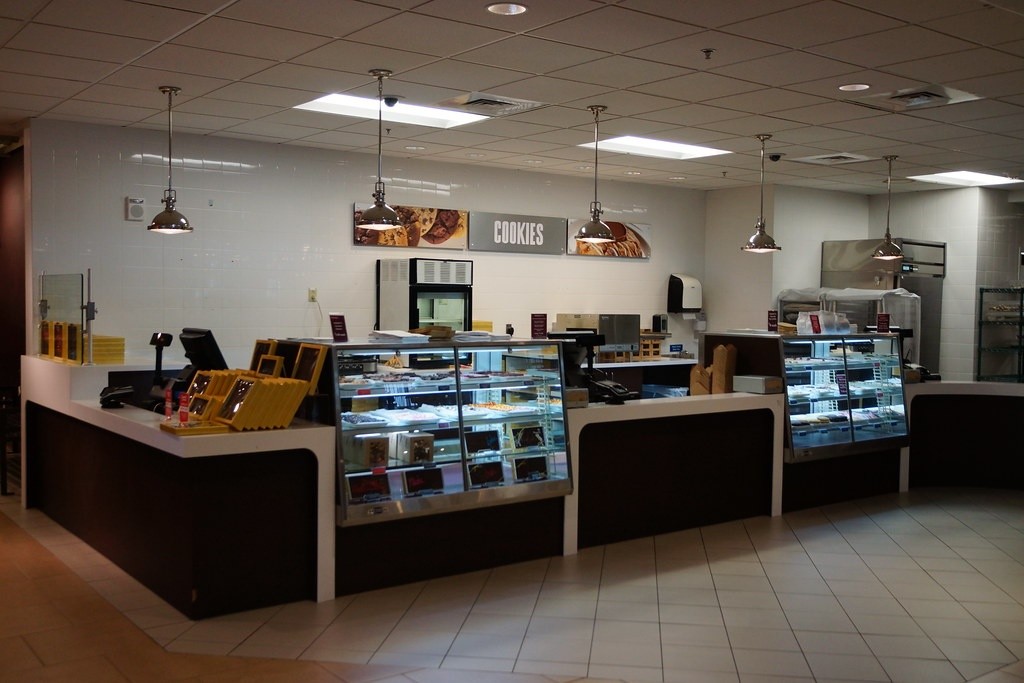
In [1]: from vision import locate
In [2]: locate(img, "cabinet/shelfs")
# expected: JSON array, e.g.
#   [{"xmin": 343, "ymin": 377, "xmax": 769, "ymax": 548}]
[
  {"xmin": 701, "ymin": 327, "xmax": 909, "ymax": 516},
  {"xmin": 288, "ymin": 336, "xmax": 573, "ymax": 598},
  {"xmin": 978, "ymin": 286, "xmax": 1024, "ymax": 384}
]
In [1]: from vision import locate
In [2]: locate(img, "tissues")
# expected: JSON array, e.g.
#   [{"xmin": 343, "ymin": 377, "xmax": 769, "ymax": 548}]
[{"xmin": 666, "ymin": 274, "xmax": 703, "ymax": 320}]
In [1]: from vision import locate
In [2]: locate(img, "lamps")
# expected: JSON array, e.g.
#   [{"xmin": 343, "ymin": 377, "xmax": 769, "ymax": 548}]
[
  {"xmin": 574, "ymin": 105, "xmax": 616, "ymax": 244},
  {"xmin": 872, "ymin": 154, "xmax": 905, "ymax": 260},
  {"xmin": 355, "ymin": 68, "xmax": 404, "ymax": 230},
  {"xmin": 146, "ymin": 86, "xmax": 193, "ymax": 234},
  {"xmin": 741, "ymin": 133, "xmax": 781, "ymax": 253}
]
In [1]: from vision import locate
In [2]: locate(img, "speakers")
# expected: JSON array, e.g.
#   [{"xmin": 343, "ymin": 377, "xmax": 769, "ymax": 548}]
[{"xmin": 126, "ymin": 196, "xmax": 145, "ymax": 221}]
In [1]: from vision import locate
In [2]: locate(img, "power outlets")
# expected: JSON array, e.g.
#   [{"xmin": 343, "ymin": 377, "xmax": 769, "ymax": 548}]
[{"xmin": 309, "ymin": 289, "xmax": 318, "ymax": 301}]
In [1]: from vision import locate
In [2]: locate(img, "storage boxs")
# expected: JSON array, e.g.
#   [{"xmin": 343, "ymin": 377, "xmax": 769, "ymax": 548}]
[
  {"xmin": 512, "ymin": 456, "xmax": 548, "ymax": 480},
  {"xmin": 689, "ymin": 344, "xmax": 736, "ymax": 396},
  {"xmin": 466, "ymin": 461, "xmax": 505, "ymax": 486},
  {"xmin": 464, "ymin": 430, "xmax": 500, "ymax": 455},
  {"xmin": 566, "ymin": 388, "xmax": 588, "ymax": 408},
  {"xmin": 344, "ymin": 433, "xmax": 390, "ymax": 468},
  {"xmin": 159, "ymin": 339, "xmax": 328, "ymax": 436},
  {"xmin": 508, "ymin": 421, "xmax": 544, "ymax": 452},
  {"xmin": 386, "ymin": 432, "xmax": 434, "ymax": 464},
  {"xmin": 347, "ymin": 473, "xmax": 392, "ymax": 503},
  {"xmin": 400, "ymin": 467, "xmax": 444, "ymax": 494},
  {"xmin": 643, "ymin": 385, "xmax": 688, "ymax": 399},
  {"xmin": 39, "ymin": 321, "xmax": 126, "ymax": 366}
]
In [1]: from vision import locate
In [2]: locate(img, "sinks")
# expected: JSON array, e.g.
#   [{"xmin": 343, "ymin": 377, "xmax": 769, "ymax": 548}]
[{"xmin": 660, "ymin": 353, "xmax": 698, "ymax": 360}]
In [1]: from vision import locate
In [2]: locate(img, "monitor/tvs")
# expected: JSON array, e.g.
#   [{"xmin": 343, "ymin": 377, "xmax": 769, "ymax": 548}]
[{"xmin": 179, "ymin": 327, "xmax": 229, "ymax": 371}]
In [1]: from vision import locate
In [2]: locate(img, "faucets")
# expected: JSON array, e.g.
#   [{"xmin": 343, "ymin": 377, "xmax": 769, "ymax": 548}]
[{"xmin": 678, "ymin": 346, "xmax": 688, "ymax": 355}]
